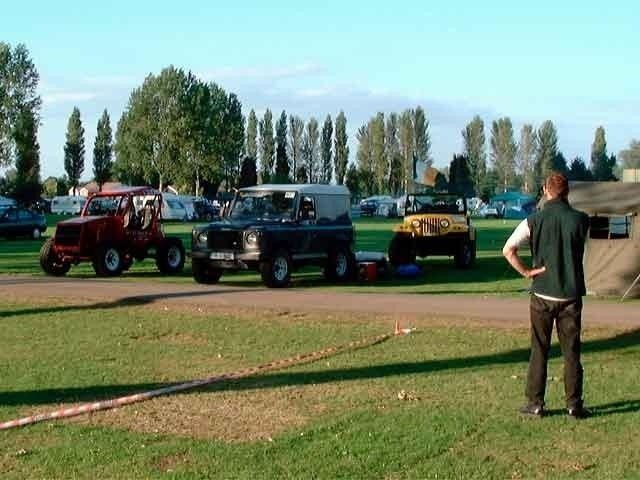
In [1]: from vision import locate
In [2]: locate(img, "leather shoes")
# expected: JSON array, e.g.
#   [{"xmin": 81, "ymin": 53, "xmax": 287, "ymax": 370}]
[
  {"xmin": 520, "ymin": 402, "xmax": 542, "ymax": 418},
  {"xmin": 568, "ymin": 405, "xmax": 596, "ymax": 420}
]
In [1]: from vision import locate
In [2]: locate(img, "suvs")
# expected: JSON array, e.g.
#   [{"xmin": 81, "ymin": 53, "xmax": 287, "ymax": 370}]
[
  {"xmin": 39, "ymin": 184, "xmax": 186, "ymax": 278},
  {"xmin": 187, "ymin": 183, "xmax": 357, "ymax": 288},
  {"xmin": 388, "ymin": 191, "xmax": 477, "ymax": 269}
]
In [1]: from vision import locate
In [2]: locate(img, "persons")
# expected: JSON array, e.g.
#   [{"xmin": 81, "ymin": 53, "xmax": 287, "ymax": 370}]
[{"xmin": 502, "ymin": 172, "xmax": 593, "ymax": 417}]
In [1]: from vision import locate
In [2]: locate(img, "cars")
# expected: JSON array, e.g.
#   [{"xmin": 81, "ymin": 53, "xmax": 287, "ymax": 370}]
[
  {"xmin": 0, "ymin": 201, "xmax": 48, "ymax": 241},
  {"xmin": 157, "ymin": 198, "xmax": 220, "ymax": 222},
  {"xmin": 359, "ymin": 194, "xmax": 394, "ymax": 214}
]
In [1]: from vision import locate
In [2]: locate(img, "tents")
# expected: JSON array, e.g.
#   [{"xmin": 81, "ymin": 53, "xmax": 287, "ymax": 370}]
[{"xmin": 535, "ymin": 178, "xmax": 639, "ymax": 299}]
